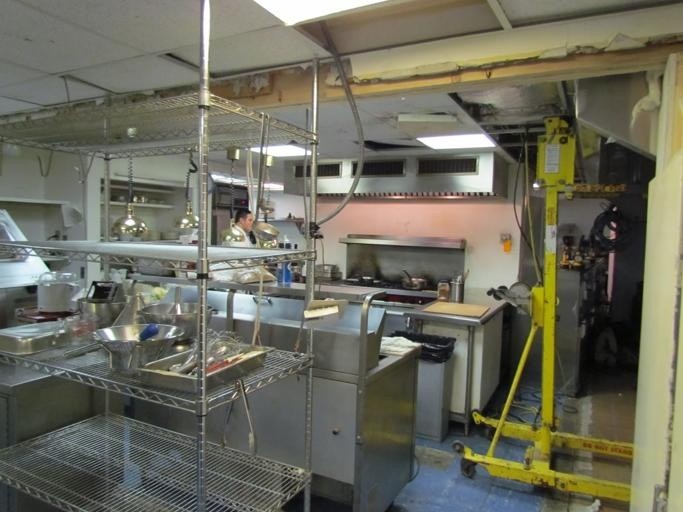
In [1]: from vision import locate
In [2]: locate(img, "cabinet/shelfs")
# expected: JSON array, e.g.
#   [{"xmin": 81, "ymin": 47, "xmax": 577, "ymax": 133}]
[{"xmin": 1, "ymin": 0, "xmax": 319, "ymax": 512}]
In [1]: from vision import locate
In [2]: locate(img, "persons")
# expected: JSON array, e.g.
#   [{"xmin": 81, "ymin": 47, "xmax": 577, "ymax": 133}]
[{"xmin": 221, "ymin": 208, "xmax": 254, "ymax": 272}]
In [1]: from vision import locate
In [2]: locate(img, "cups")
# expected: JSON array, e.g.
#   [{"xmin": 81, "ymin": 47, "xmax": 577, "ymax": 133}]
[{"xmin": 451, "ymin": 282, "xmax": 464, "ymax": 302}]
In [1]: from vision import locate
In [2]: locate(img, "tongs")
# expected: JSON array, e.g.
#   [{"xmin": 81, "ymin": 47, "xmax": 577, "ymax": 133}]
[{"xmin": 220, "ymin": 379, "xmax": 258, "ymax": 455}]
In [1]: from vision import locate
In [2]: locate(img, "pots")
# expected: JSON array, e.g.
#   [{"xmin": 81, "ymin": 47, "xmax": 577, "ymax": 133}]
[
  {"xmin": 36, "ymin": 271, "xmax": 80, "ymax": 313},
  {"xmin": 136, "ymin": 301, "xmax": 218, "ymax": 342},
  {"xmin": 401, "ymin": 269, "xmax": 429, "ymax": 289}
]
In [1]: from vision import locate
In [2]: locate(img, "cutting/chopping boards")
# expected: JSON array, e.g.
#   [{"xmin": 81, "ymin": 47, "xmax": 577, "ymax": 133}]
[{"xmin": 422, "ymin": 302, "xmax": 491, "ymax": 318}]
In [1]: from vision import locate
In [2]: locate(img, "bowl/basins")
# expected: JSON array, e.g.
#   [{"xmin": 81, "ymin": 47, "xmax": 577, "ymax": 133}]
[
  {"xmin": 90, "ymin": 323, "xmax": 184, "ymax": 373},
  {"xmin": 77, "ymin": 296, "xmax": 129, "ymax": 328}
]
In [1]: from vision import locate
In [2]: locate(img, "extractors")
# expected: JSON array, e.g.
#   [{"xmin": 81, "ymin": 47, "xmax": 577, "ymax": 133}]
[{"xmin": 283, "ymin": 152, "xmax": 509, "ymax": 198}]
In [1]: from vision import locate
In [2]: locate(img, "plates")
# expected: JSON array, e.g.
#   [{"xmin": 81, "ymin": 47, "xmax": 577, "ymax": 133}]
[
  {"xmin": 161, "ymin": 230, "xmax": 179, "ymax": 240},
  {"xmin": 149, "ymin": 230, "xmax": 161, "ymax": 240}
]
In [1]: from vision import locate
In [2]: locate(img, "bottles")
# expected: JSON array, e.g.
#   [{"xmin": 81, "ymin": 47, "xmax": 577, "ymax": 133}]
[
  {"xmin": 437, "ymin": 280, "xmax": 450, "ymax": 301},
  {"xmin": 291, "ymin": 244, "xmax": 302, "ymax": 282},
  {"xmin": 282, "ymin": 243, "xmax": 292, "ymax": 283},
  {"xmin": 277, "ymin": 243, "xmax": 285, "ymax": 282}
]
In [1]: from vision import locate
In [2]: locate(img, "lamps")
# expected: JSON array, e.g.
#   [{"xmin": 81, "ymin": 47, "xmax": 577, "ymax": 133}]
[
  {"xmin": 219, "ymin": 148, "xmax": 244, "ymax": 242},
  {"xmin": 171, "ymin": 150, "xmax": 200, "ymax": 228},
  {"xmin": 111, "ymin": 128, "xmax": 149, "ymax": 239},
  {"xmin": 258, "ymin": 155, "xmax": 279, "ymax": 249}
]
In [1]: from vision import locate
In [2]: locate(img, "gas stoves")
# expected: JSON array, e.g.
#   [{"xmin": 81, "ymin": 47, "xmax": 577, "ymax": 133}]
[{"xmin": 337, "ymin": 277, "xmax": 436, "ymax": 291}]
